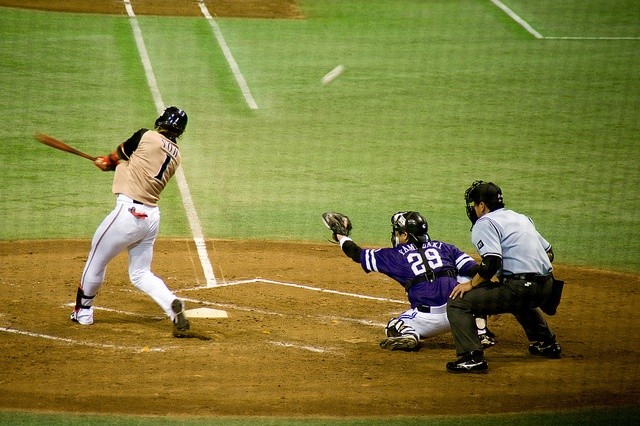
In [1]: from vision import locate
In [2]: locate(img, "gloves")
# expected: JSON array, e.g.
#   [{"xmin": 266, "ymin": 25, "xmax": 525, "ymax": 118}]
[
  {"xmin": 96, "ymin": 154, "xmax": 117, "ymax": 166},
  {"xmin": 98, "ymin": 160, "xmax": 121, "ymax": 172}
]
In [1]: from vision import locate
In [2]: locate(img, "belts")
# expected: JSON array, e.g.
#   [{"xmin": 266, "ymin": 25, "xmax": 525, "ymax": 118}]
[{"xmin": 514, "ymin": 273, "xmax": 550, "ymax": 282}]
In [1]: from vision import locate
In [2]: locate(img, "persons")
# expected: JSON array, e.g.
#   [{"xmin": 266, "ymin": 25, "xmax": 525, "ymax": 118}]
[
  {"xmin": 322, "ymin": 211, "xmax": 495, "ymax": 349},
  {"xmin": 71, "ymin": 105, "xmax": 190, "ymax": 339},
  {"xmin": 446, "ymin": 179, "xmax": 564, "ymax": 373}
]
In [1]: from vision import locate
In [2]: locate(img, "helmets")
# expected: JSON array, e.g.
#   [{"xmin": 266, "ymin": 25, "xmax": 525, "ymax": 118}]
[
  {"xmin": 390, "ymin": 211, "xmax": 428, "ymax": 248},
  {"xmin": 464, "ymin": 180, "xmax": 503, "ymax": 231},
  {"xmin": 155, "ymin": 106, "xmax": 188, "ymax": 137}
]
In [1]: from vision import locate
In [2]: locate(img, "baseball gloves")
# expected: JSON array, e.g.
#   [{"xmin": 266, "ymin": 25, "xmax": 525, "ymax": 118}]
[{"xmin": 322, "ymin": 210, "xmax": 352, "ymax": 240}]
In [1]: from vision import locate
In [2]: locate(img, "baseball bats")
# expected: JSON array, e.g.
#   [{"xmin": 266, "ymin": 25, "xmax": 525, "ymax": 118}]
[{"xmin": 35, "ymin": 132, "xmax": 98, "ymax": 161}]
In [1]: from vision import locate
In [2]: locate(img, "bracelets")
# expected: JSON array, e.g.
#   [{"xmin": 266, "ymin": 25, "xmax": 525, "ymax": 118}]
[{"xmin": 470, "ymin": 280, "xmax": 475, "ymax": 288}]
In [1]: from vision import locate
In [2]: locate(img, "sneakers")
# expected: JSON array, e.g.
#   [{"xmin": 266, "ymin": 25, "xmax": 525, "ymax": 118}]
[
  {"xmin": 530, "ymin": 340, "xmax": 561, "ymax": 357},
  {"xmin": 171, "ymin": 299, "xmax": 189, "ymax": 332},
  {"xmin": 380, "ymin": 332, "xmax": 420, "ymax": 351},
  {"xmin": 478, "ymin": 328, "xmax": 496, "ymax": 348},
  {"xmin": 446, "ymin": 351, "xmax": 489, "ymax": 373},
  {"xmin": 71, "ymin": 308, "xmax": 94, "ymax": 325}
]
